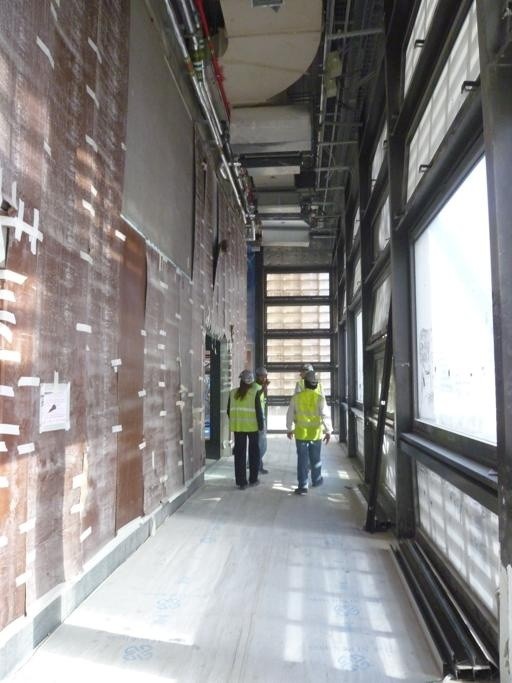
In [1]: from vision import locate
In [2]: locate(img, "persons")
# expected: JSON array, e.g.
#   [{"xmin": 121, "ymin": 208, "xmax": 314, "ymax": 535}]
[
  {"xmin": 294, "ymin": 362, "xmax": 325, "ymax": 398},
  {"xmin": 251, "ymin": 367, "xmax": 271, "ymax": 474},
  {"xmin": 285, "ymin": 370, "xmax": 331, "ymax": 495},
  {"xmin": 227, "ymin": 369, "xmax": 263, "ymax": 488}
]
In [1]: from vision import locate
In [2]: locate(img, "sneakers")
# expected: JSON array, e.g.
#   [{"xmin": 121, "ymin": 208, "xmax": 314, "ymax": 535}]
[
  {"xmin": 240, "ymin": 479, "xmax": 260, "ymax": 489},
  {"xmin": 295, "ymin": 488, "xmax": 306, "ymax": 493},
  {"xmin": 313, "ymin": 477, "xmax": 322, "ymax": 486},
  {"xmin": 259, "ymin": 468, "xmax": 268, "ymax": 474}
]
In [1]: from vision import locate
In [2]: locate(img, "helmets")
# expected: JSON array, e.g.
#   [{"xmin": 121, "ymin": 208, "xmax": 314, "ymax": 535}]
[
  {"xmin": 256, "ymin": 367, "xmax": 267, "ymax": 375},
  {"xmin": 306, "ymin": 372, "xmax": 318, "ymax": 382},
  {"xmin": 239, "ymin": 369, "xmax": 255, "ymax": 382},
  {"xmin": 303, "ymin": 364, "xmax": 313, "ymax": 371}
]
